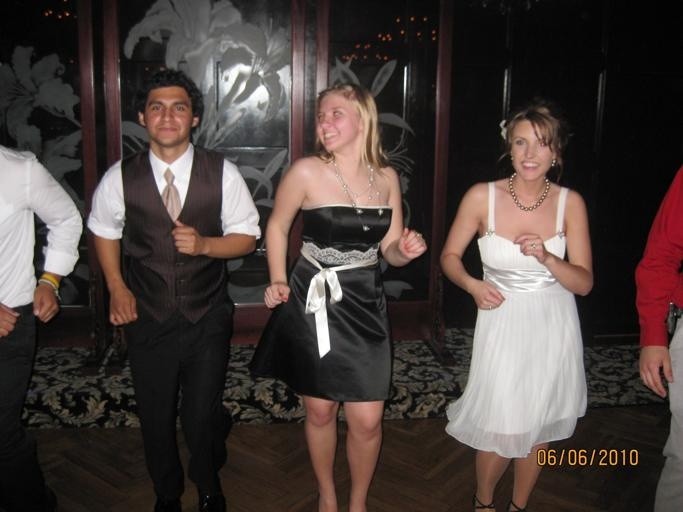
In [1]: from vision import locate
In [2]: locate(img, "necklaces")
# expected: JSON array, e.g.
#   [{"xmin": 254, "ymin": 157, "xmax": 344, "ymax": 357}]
[
  {"xmin": 330, "ymin": 156, "xmax": 385, "ymax": 233},
  {"xmin": 509, "ymin": 173, "xmax": 551, "ymax": 212}
]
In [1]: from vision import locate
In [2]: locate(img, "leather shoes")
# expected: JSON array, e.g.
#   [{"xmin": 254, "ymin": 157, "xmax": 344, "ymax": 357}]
[
  {"xmin": 155, "ymin": 480, "xmax": 184, "ymax": 512},
  {"xmin": 198, "ymin": 473, "xmax": 226, "ymax": 512}
]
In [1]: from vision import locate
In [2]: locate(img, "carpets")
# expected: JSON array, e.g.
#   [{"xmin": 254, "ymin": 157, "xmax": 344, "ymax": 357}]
[
  {"xmin": 13, "ymin": 332, "xmax": 461, "ymax": 431},
  {"xmin": 444, "ymin": 323, "xmax": 670, "ymax": 408}
]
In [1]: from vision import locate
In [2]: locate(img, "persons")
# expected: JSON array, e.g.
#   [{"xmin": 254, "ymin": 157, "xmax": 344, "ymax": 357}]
[
  {"xmin": 634, "ymin": 164, "xmax": 683, "ymax": 512},
  {"xmin": 0, "ymin": 145, "xmax": 84, "ymax": 512},
  {"xmin": 85, "ymin": 68, "xmax": 262, "ymax": 511},
  {"xmin": 247, "ymin": 83, "xmax": 428, "ymax": 512},
  {"xmin": 439, "ymin": 107, "xmax": 594, "ymax": 512}
]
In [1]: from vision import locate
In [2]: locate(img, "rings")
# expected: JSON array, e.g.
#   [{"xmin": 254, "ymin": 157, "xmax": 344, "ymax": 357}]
[
  {"xmin": 415, "ymin": 231, "xmax": 424, "ymax": 238},
  {"xmin": 531, "ymin": 243, "xmax": 536, "ymax": 249}
]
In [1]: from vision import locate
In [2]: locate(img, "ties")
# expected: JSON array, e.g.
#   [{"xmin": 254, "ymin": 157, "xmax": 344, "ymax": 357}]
[{"xmin": 162, "ymin": 168, "xmax": 182, "ymax": 221}]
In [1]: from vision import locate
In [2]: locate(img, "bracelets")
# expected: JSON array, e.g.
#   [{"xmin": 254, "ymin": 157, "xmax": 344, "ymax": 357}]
[
  {"xmin": 41, "ymin": 274, "xmax": 60, "ymax": 288},
  {"xmin": 37, "ymin": 279, "xmax": 61, "ymax": 300}
]
